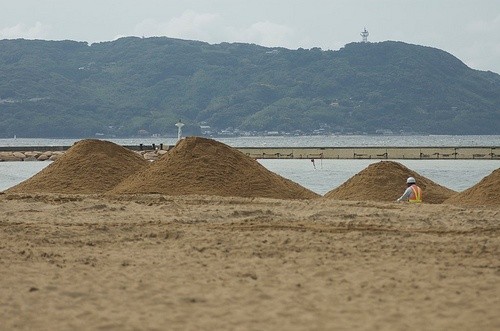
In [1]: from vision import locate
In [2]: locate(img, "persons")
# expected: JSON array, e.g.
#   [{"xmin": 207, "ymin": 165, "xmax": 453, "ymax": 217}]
[{"xmin": 396, "ymin": 176, "xmax": 423, "ymax": 204}]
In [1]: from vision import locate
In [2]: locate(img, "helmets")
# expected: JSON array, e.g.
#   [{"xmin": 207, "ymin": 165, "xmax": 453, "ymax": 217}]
[{"xmin": 406, "ymin": 176, "xmax": 415, "ymax": 184}]
